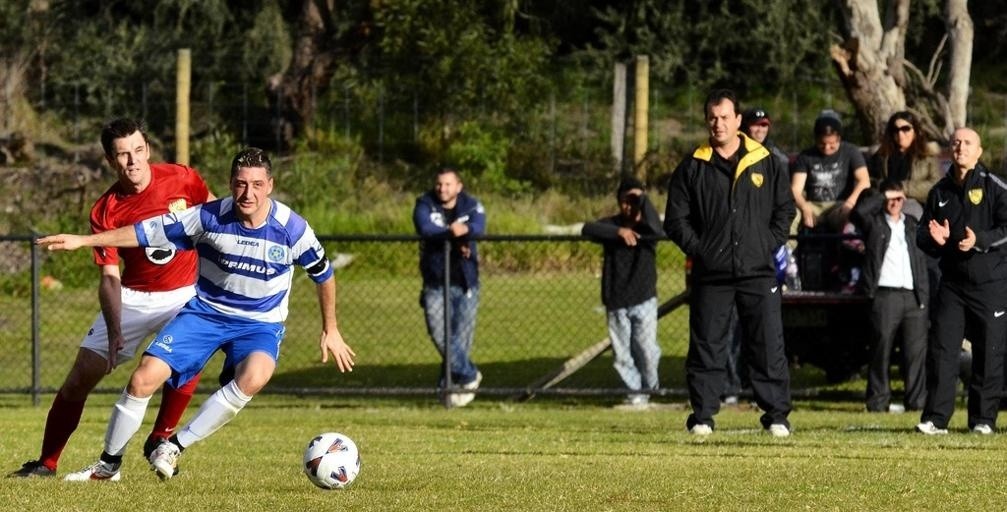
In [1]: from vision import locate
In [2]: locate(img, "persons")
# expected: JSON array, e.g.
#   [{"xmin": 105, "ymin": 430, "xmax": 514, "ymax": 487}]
[
  {"xmin": 411, "ymin": 169, "xmax": 484, "ymax": 409},
  {"xmin": 579, "ymin": 173, "xmax": 664, "ymax": 409},
  {"xmin": 789, "ymin": 112, "xmax": 870, "ymax": 251},
  {"xmin": 4, "ymin": 117, "xmax": 219, "ymax": 478},
  {"xmin": 32, "ymin": 148, "xmax": 355, "ymax": 484},
  {"xmin": 740, "ymin": 109, "xmax": 791, "ymax": 185},
  {"xmin": 916, "ymin": 128, "xmax": 1006, "ymax": 435},
  {"xmin": 667, "ymin": 87, "xmax": 797, "ymax": 440},
  {"xmin": 859, "ymin": 177, "xmax": 930, "ymax": 412},
  {"xmin": 868, "ymin": 111, "xmax": 943, "ymax": 222}
]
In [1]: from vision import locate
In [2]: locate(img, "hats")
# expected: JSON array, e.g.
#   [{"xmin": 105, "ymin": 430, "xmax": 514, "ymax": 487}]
[{"xmin": 748, "ymin": 109, "xmax": 771, "ymax": 126}]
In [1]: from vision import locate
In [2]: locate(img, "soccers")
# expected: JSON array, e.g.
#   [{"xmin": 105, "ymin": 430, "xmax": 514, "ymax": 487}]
[{"xmin": 303, "ymin": 433, "xmax": 360, "ymax": 491}]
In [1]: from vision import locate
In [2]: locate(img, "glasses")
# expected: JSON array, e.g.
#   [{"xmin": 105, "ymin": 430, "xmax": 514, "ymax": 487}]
[{"xmin": 894, "ymin": 125, "xmax": 913, "ymax": 133}]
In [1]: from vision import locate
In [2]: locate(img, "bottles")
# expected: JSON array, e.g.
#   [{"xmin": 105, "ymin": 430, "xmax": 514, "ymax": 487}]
[{"xmin": 785, "ymin": 257, "xmax": 801, "ymax": 293}]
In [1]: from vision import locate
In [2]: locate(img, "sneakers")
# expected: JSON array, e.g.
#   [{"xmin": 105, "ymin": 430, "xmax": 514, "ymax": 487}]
[
  {"xmin": 974, "ymin": 423, "xmax": 992, "ymax": 435},
  {"xmin": 144, "ymin": 434, "xmax": 181, "ymax": 480},
  {"xmin": 14, "ymin": 460, "xmax": 56, "ymax": 478},
  {"xmin": 691, "ymin": 424, "xmax": 713, "ymax": 435},
  {"xmin": 915, "ymin": 420, "xmax": 948, "ymax": 436},
  {"xmin": 64, "ymin": 458, "xmax": 121, "ymax": 483},
  {"xmin": 770, "ymin": 424, "xmax": 790, "ymax": 437},
  {"xmin": 450, "ymin": 371, "xmax": 483, "ymax": 408}
]
